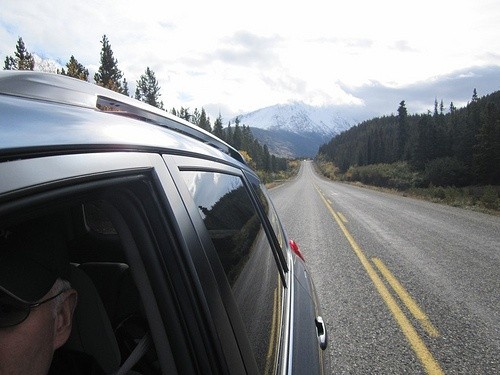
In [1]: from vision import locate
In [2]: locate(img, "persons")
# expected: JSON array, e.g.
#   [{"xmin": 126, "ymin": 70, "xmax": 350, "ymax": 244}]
[{"xmin": 0, "ymin": 217, "xmax": 110, "ymax": 375}]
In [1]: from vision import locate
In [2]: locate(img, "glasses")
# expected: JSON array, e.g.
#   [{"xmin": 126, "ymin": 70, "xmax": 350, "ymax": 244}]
[{"xmin": 0, "ymin": 288, "xmax": 70, "ymax": 328}]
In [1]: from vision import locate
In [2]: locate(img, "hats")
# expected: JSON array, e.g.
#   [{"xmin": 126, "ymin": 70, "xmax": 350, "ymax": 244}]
[{"xmin": 0, "ymin": 230, "xmax": 72, "ymax": 305}]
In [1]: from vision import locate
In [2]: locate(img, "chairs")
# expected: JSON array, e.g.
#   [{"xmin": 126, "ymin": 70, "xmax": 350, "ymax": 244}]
[
  {"xmin": 59, "ymin": 265, "xmax": 120, "ymax": 372},
  {"xmin": 82, "ymin": 261, "xmax": 141, "ymax": 338}
]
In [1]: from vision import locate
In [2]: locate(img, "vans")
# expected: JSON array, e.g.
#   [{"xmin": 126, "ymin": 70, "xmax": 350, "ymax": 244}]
[{"xmin": 0, "ymin": 65, "xmax": 332, "ymax": 375}]
